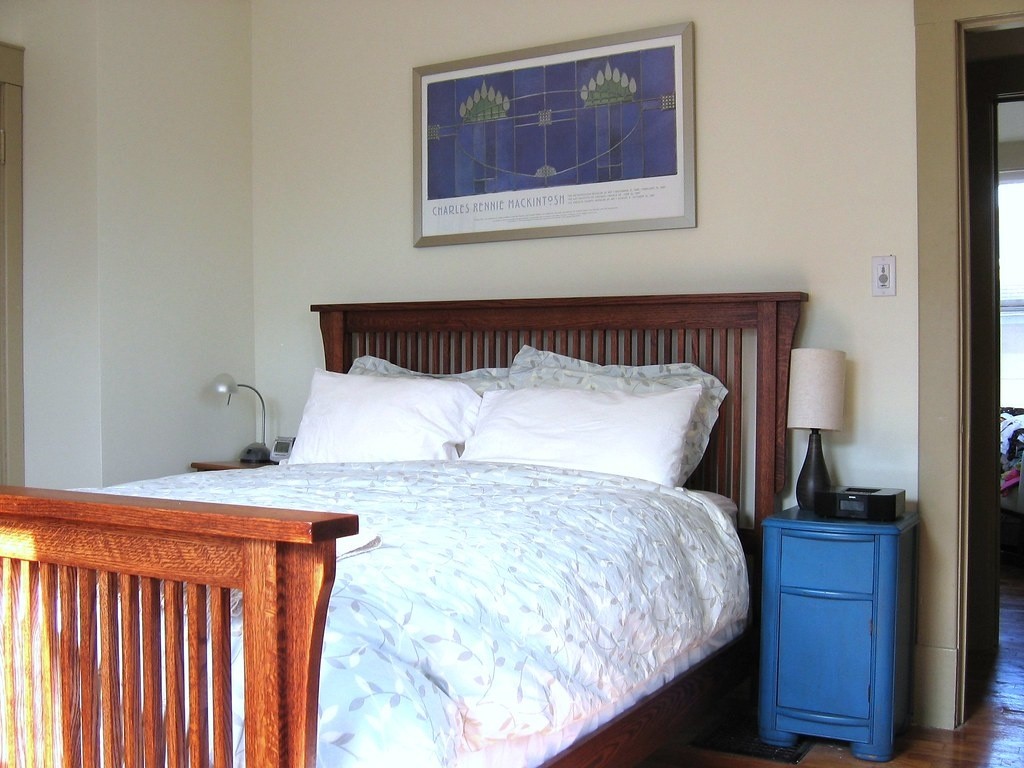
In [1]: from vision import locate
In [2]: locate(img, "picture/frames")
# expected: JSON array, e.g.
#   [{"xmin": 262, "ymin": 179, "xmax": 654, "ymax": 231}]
[{"xmin": 413, "ymin": 22, "xmax": 697, "ymax": 247}]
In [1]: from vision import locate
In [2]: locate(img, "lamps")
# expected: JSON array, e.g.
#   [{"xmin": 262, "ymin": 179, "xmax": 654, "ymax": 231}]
[
  {"xmin": 787, "ymin": 348, "xmax": 846, "ymax": 510},
  {"xmin": 213, "ymin": 374, "xmax": 270, "ymax": 463}
]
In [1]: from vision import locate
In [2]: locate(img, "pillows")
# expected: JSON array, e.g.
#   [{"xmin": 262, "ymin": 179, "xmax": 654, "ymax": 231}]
[{"xmin": 279, "ymin": 344, "xmax": 729, "ymax": 490}]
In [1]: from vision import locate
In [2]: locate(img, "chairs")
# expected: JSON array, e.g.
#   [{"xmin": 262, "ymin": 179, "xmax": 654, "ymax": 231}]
[{"xmin": 1000, "ymin": 450, "xmax": 1024, "ymax": 569}]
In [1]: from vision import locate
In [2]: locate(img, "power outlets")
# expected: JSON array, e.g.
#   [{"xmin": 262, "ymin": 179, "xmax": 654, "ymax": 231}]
[{"xmin": 871, "ymin": 256, "xmax": 896, "ymax": 296}]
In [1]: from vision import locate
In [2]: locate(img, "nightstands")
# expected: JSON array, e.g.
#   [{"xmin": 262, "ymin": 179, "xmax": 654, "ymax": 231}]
[
  {"xmin": 758, "ymin": 505, "xmax": 920, "ymax": 762},
  {"xmin": 191, "ymin": 461, "xmax": 270, "ymax": 471}
]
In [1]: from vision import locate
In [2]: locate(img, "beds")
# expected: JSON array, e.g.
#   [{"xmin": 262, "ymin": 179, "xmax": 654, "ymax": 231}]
[{"xmin": 0, "ymin": 292, "xmax": 809, "ymax": 768}]
